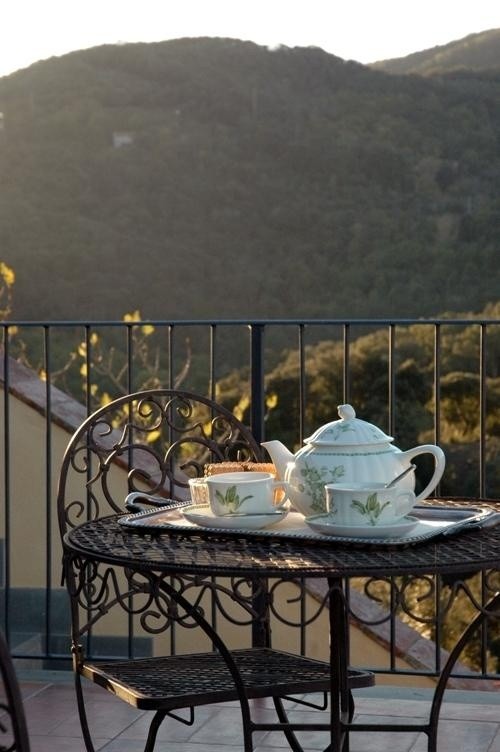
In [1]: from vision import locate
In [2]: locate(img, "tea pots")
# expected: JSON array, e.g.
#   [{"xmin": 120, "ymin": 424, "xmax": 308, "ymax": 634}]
[{"xmin": 259, "ymin": 402, "xmax": 448, "ymax": 518}]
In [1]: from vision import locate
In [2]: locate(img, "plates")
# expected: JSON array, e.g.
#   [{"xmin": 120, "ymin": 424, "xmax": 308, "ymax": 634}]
[
  {"xmin": 180, "ymin": 503, "xmax": 291, "ymax": 530},
  {"xmin": 304, "ymin": 511, "xmax": 422, "ymax": 538}
]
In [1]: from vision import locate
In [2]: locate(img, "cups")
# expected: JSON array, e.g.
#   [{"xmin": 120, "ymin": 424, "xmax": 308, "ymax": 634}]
[
  {"xmin": 323, "ymin": 480, "xmax": 417, "ymax": 526},
  {"xmin": 204, "ymin": 471, "xmax": 292, "ymax": 515},
  {"xmin": 188, "ymin": 477, "xmax": 209, "ymax": 505}
]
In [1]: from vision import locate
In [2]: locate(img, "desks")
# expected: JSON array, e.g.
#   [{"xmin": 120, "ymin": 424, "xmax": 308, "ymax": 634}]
[{"xmin": 62, "ymin": 496, "xmax": 500, "ymax": 752}]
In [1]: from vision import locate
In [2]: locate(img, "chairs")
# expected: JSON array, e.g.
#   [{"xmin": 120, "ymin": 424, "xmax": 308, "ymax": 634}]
[{"xmin": 57, "ymin": 390, "xmax": 375, "ymax": 752}]
